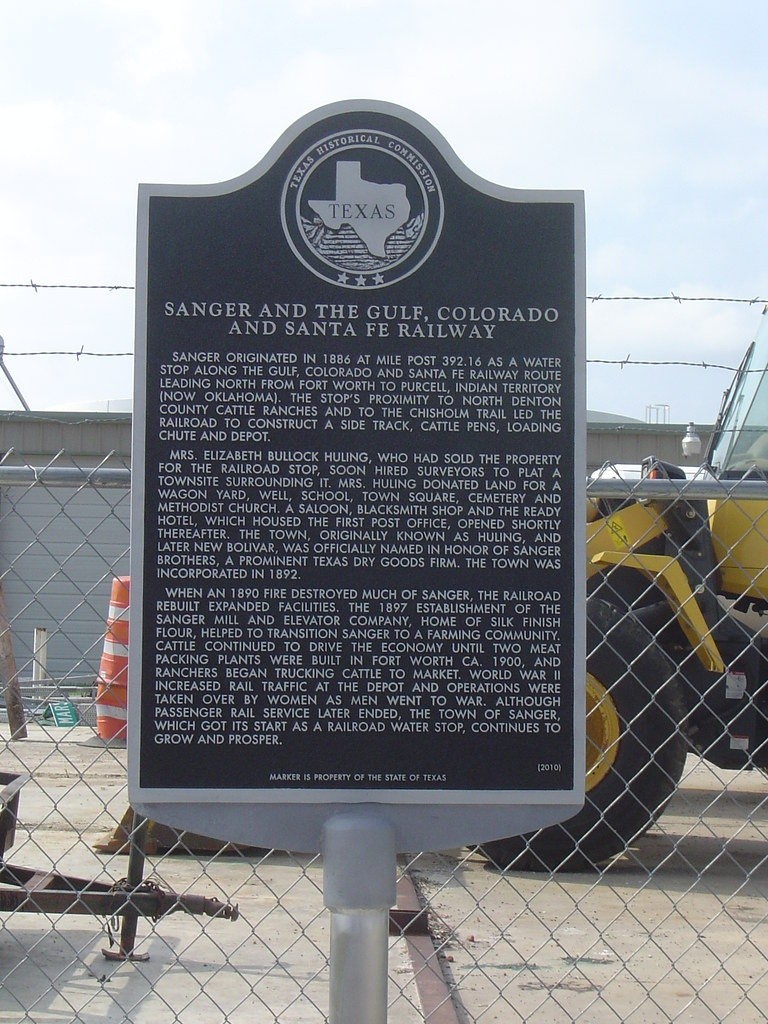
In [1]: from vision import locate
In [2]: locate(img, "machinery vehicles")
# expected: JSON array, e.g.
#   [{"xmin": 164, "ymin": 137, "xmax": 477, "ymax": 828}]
[{"xmin": 89, "ymin": 301, "xmax": 768, "ymax": 873}]
